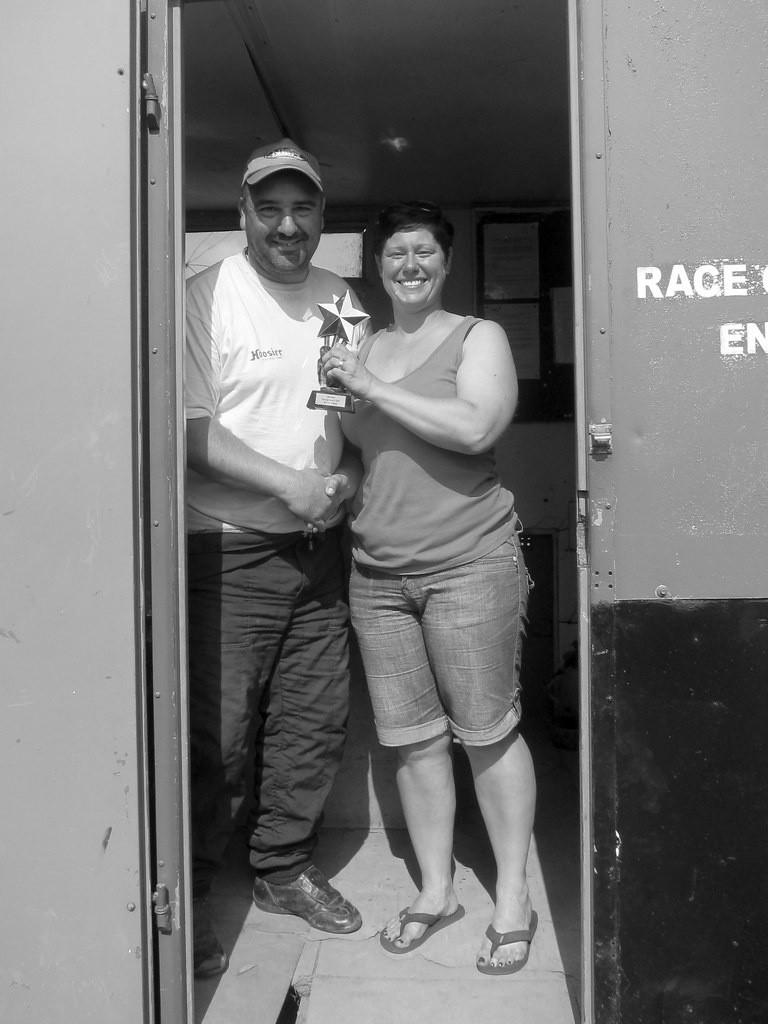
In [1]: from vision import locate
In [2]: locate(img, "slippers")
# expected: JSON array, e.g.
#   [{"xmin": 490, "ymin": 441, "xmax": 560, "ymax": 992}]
[
  {"xmin": 381, "ymin": 897, "xmax": 466, "ymax": 954},
  {"xmin": 477, "ymin": 908, "xmax": 538, "ymax": 975}
]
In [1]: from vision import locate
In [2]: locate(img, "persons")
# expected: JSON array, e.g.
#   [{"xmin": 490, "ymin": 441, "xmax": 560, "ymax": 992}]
[
  {"xmin": 314, "ymin": 200, "xmax": 538, "ymax": 974},
  {"xmin": 186, "ymin": 139, "xmax": 372, "ymax": 979}
]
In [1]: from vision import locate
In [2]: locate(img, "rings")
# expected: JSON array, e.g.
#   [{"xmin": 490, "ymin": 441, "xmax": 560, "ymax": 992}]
[{"xmin": 339, "ymin": 361, "xmax": 343, "ymax": 370}]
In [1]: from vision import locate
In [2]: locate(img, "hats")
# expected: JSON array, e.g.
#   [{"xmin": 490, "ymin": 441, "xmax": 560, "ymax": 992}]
[{"xmin": 241, "ymin": 138, "xmax": 323, "ymax": 194}]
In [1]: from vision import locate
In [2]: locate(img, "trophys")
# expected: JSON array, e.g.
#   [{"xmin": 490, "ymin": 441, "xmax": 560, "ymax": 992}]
[{"xmin": 306, "ymin": 289, "xmax": 371, "ymax": 413}]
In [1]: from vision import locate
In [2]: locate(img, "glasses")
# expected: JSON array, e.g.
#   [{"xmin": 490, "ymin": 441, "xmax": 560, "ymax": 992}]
[{"xmin": 379, "ymin": 201, "xmax": 445, "ymax": 226}]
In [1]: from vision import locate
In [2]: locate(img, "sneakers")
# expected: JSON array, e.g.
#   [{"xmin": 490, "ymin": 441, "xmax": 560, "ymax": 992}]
[
  {"xmin": 194, "ymin": 895, "xmax": 226, "ymax": 979},
  {"xmin": 252, "ymin": 864, "xmax": 363, "ymax": 935}
]
